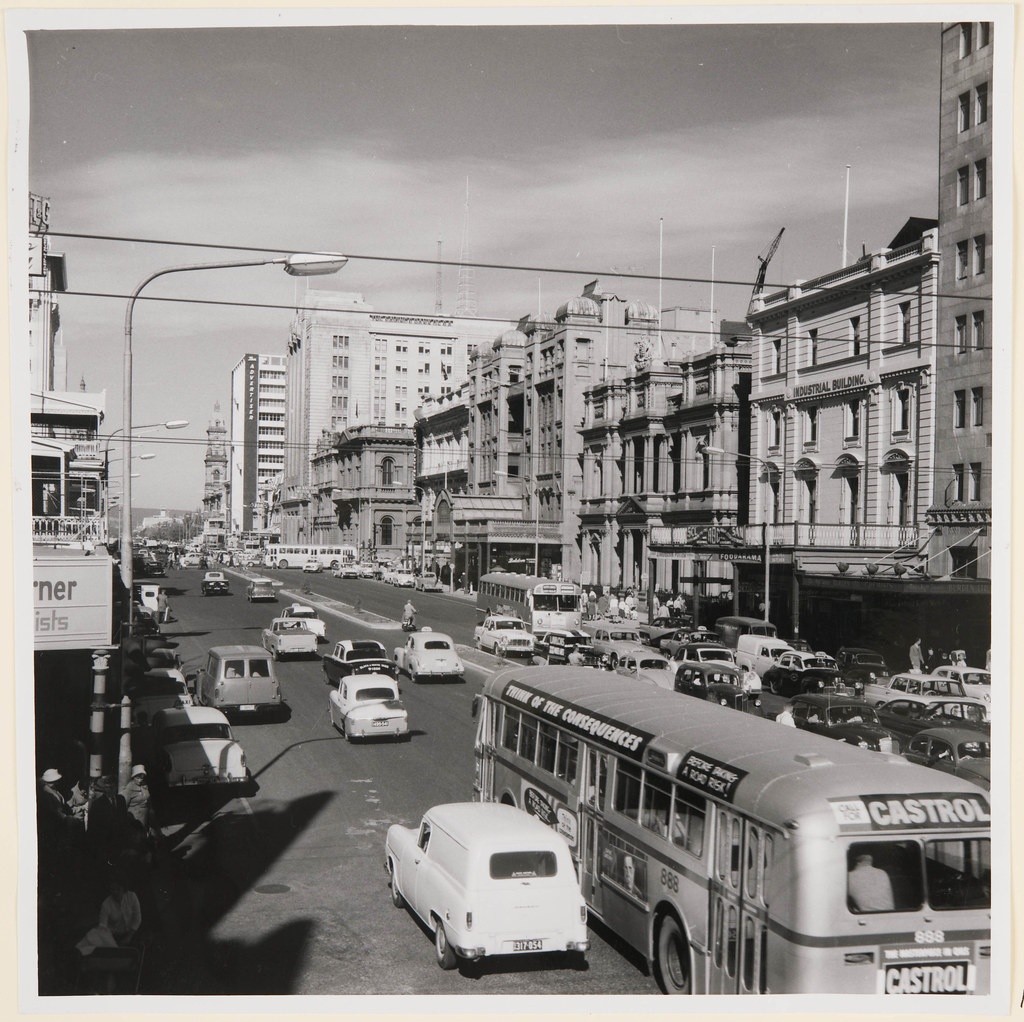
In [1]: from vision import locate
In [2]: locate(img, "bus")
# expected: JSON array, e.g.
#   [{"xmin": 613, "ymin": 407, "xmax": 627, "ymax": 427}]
[
  {"xmin": 472, "ymin": 664, "xmax": 991, "ymax": 995},
  {"xmin": 476, "ymin": 571, "xmax": 583, "ymax": 633}
]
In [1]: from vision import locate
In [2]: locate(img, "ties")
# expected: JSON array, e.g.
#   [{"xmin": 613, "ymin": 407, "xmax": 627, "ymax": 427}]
[{"xmin": 111, "ymin": 797, "xmax": 117, "ymax": 809}]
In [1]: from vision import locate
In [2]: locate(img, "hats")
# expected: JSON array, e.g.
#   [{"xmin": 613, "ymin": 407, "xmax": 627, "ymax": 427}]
[
  {"xmin": 43, "ymin": 768, "xmax": 61, "ymax": 782},
  {"xmin": 130, "ymin": 764, "xmax": 147, "ymax": 779}
]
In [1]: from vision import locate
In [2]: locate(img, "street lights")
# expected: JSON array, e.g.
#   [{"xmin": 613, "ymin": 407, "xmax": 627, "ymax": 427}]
[
  {"xmin": 118, "ymin": 251, "xmax": 348, "ymax": 791},
  {"xmin": 493, "ymin": 471, "xmax": 539, "ymax": 576},
  {"xmin": 104, "ymin": 420, "xmax": 189, "ymax": 547},
  {"xmin": 702, "ymin": 447, "xmax": 770, "ymax": 620}
]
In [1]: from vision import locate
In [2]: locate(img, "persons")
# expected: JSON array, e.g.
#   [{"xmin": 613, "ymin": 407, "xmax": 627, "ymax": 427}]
[
  {"xmin": 156, "ymin": 589, "xmax": 170, "ymax": 623},
  {"xmin": 619, "ymin": 855, "xmax": 644, "ymax": 901},
  {"xmin": 402, "ymin": 599, "xmax": 416, "ymax": 627},
  {"xmin": 909, "ymin": 637, "xmax": 924, "ymax": 668},
  {"xmin": 35, "ymin": 710, "xmax": 149, "ymax": 995},
  {"xmin": 774, "ymin": 702, "xmax": 795, "ymax": 728},
  {"xmin": 579, "ymin": 587, "xmax": 687, "ymax": 624},
  {"xmin": 849, "ymin": 848, "xmax": 898, "ymax": 912}
]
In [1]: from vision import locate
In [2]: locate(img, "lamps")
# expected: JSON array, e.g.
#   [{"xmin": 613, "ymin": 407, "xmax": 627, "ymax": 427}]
[
  {"xmin": 865, "ymin": 563, "xmax": 879, "ymax": 578},
  {"xmin": 893, "ymin": 562, "xmax": 908, "ymax": 579},
  {"xmin": 836, "ymin": 562, "xmax": 849, "ymax": 576}
]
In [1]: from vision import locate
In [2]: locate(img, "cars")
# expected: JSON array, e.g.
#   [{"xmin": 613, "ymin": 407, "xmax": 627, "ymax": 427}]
[
  {"xmin": 785, "ymin": 693, "xmax": 904, "ymax": 754},
  {"xmin": 385, "ymin": 802, "xmax": 590, "ymax": 971},
  {"xmin": 196, "ymin": 645, "xmax": 282, "ymax": 714},
  {"xmin": 473, "ymin": 615, "xmax": 538, "ymax": 656},
  {"xmin": 900, "ymin": 728, "xmax": 990, "ymax": 792},
  {"xmin": 139, "ymin": 543, "xmax": 443, "ymax": 603},
  {"xmin": 531, "ymin": 627, "xmax": 661, "ymax": 670},
  {"xmin": 393, "ymin": 631, "xmax": 464, "ymax": 684},
  {"xmin": 321, "ymin": 639, "xmax": 400, "ymax": 688},
  {"xmin": 639, "ymin": 616, "xmax": 891, "ymax": 718},
  {"xmin": 123, "ymin": 601, "xmax": 194, "ymax": 725},
  {"xmin": 328, "ymin": 674, "xmax": 409, "ymax": 741},
  {"xmin": 151, "ymin": 706, "xmax": 249, "ymax": 787},
  {"xmin": 864, "ymin": 666, "xmax": 991, "ymax": 740},
  {"xmin": 262, "ymin": 606, "xmax": 328, "ymax": 659}
]
